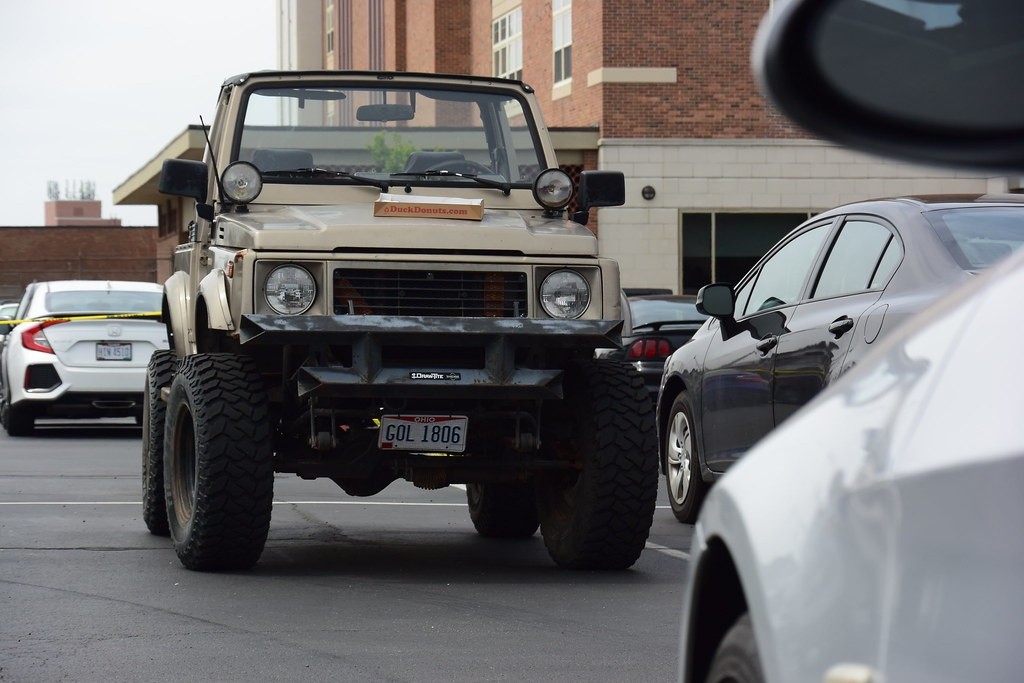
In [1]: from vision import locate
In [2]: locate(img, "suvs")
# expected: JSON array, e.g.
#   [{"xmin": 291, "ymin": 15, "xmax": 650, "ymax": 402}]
[{"xmin": 142, "ymin": 71, "xmax": 660, "ymax": 572}]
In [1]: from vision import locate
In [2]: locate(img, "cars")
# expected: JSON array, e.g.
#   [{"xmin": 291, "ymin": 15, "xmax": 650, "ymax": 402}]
[
  {"xmin": 628, "ymin": 296, "xmax": 712, "ymax": 406},
  {"xmin": 655, "ymin": 193, "xmax": 1024, "ymax": 522},
  {"xmin": 0, "ymin": 280, "xmax": 171, "ymax": 437},
  {"xmin": 680, "ymin": 0, "xmax": 1024, "ymax": 680}
]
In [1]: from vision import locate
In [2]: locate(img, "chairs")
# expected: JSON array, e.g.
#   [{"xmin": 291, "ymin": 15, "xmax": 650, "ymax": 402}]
[
  {"xmin": 252, "ymin": 149, "xmax": 313, "ymax": 175},
  {"xmin": 404, "ymin": 152, "xmax": 469, "ymax": 174}
]
